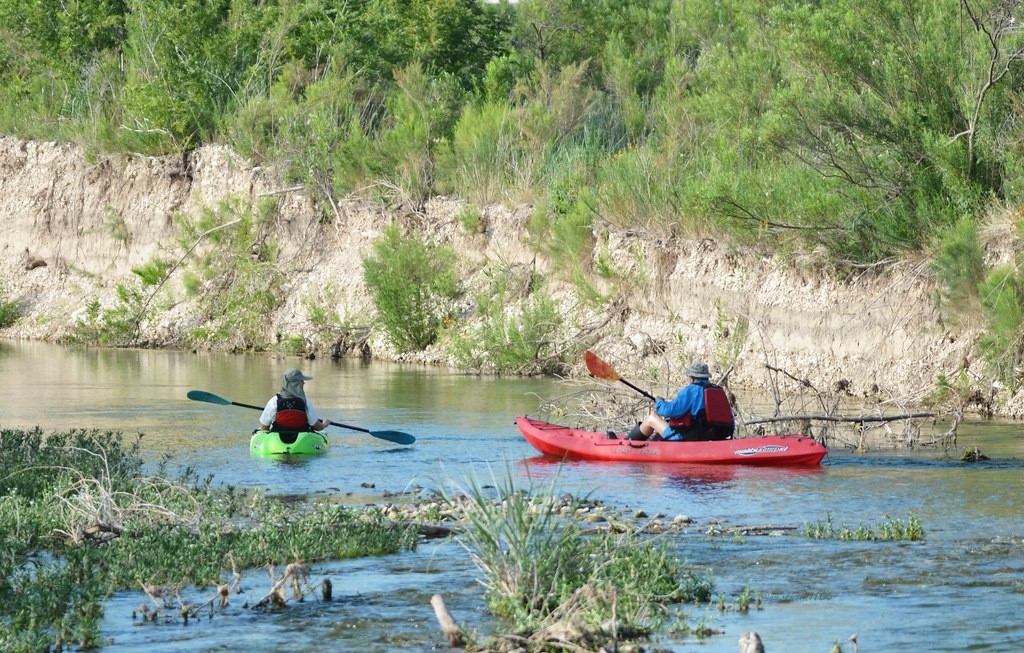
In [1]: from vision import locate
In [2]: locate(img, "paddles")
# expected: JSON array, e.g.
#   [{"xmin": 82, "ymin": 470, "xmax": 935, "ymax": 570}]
[
  {"xmin": 187, "ymin": 390, "xmax": 416, "ymax": 445},
  {"xmin": 585, "ymin": 349, "xmax": 658, "ymax": 403}
]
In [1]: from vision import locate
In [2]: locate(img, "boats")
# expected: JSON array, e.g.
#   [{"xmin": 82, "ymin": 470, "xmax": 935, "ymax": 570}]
[
  {"xmin": 249, "ymin": 426, "xmax": 329, "ymax": 461},
  {"xmin": 514, "ymin": 415, "xmax": 829, "ymax": 467}
]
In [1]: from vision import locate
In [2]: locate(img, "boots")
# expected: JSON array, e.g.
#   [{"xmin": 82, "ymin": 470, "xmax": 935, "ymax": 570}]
[
  {"xmin": 607, "ymin": 422, "xmax": 649, "ymax": 440},
  {"xmin": 652, "ymin": 433, "xmax": 666, "ymax": 441}
]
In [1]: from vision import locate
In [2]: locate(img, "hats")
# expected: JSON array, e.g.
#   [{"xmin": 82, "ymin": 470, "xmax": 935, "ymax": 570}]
[
  {"xmin": 687, "ymin": 360, "xmax": 712, "ymax": 379},
  {"xmin": 278, "ymin": 368, "xmax": 313, "ymax": 411}
]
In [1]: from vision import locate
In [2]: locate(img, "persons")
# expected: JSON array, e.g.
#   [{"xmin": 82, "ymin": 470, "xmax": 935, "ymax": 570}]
[
  {"xmin": 258, "ymin": 370, "xmax": 331, "ymax": 432},
  {"xmin": 606, "ymin": 361, "xmax": 734, "ymax": 442}
]
